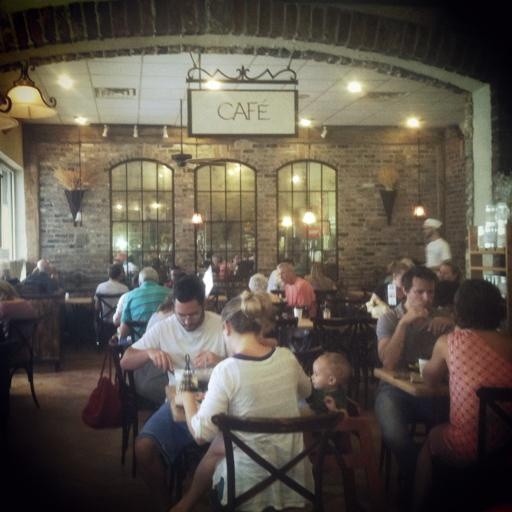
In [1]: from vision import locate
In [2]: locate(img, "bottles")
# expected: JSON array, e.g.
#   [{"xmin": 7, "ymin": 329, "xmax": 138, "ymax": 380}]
[
  {"xmin": 276, "ymin": 285, "xmax": 283, "ymax": 303},
  {"xmin": 323, "ymin": 300, "xmax": 330, "ymax": 319},
  {"xmin": 175, "ymin": 355, "xmax": 199, "ymax": 398},
  {"xmin": 484, "ymin": 274, "xmax": 506, "ymax": 298}
]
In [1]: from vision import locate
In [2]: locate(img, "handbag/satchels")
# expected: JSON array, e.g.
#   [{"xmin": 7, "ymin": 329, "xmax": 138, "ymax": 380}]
[{"xmin": 82, "ymin": 377, "xmax": 123, "ymax": 428}]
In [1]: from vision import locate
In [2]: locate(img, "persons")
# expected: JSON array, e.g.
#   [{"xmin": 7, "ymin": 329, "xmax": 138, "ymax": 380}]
[
  {"xmin": 95, "ymin": 217, "xmax": 512, "ymax": 512},
  {"xmin": 0, "ymin": 279, "xmax": 34, "ymax": 368},
  {"xmin": 21, "ymin": 259, "xmax": 59, "ymax": 300}
]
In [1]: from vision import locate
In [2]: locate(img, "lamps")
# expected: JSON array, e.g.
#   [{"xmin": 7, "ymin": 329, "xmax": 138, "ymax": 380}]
[
  {"xmin": 102, "ymin": 124, "xmax": 112, "ymax": 138},
  {"xmin": 133, "ymin": 125, "xmax": 139, "ymax": 139},
  {"xmin": 162, "ymin": 126, "xmax": 168, "ymax": 138},
  {"xmin": 192, "ymin": 170, "xmax": 203, "ymax": 223},
  {"xmin": 414, "ymin": 128, "xmax": 425, "ymax": 218},
  {"xmin": 0, "ymin": 58, "xmax": 56, "ymax": 118}
]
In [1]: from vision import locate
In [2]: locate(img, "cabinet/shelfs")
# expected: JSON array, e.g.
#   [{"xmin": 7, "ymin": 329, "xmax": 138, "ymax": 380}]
[{"xmin": 468, "ymin": 249, "xmax": 510, "ymax": 334}]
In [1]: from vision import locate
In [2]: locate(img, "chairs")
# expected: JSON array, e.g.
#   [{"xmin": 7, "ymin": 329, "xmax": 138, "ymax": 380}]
[
  {"xmin": 3, "ymin": 276, "xmax": 508, "ymax": 409},
  {"xmin": 212, "ymin": 412, "xmax": 346, "ymax": 510},
  {"xmin": 110, "ymin": 343, "xmax": 166, "ymax": 474},
  {"xmin": 428, "ymin": 380, "xmax": 510, "ymax": 510}
]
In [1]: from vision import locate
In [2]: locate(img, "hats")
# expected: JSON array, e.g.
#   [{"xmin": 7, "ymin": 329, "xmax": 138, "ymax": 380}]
[{"xmin": 425, "ymin": 218, "xmax": 442, "ymax": 228}]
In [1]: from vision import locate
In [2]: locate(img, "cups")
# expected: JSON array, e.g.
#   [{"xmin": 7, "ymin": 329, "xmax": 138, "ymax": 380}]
[
  {"xmin": 418, "ymin": 357, "xmax": 429, "ymax": 377},
  {"xmin": 483, "ymin": 202, "xmax": 507, "ymax": 250},
  {"xmin": 294, "ymin": 308, "xmax": 302, "ymax": 321}
]
